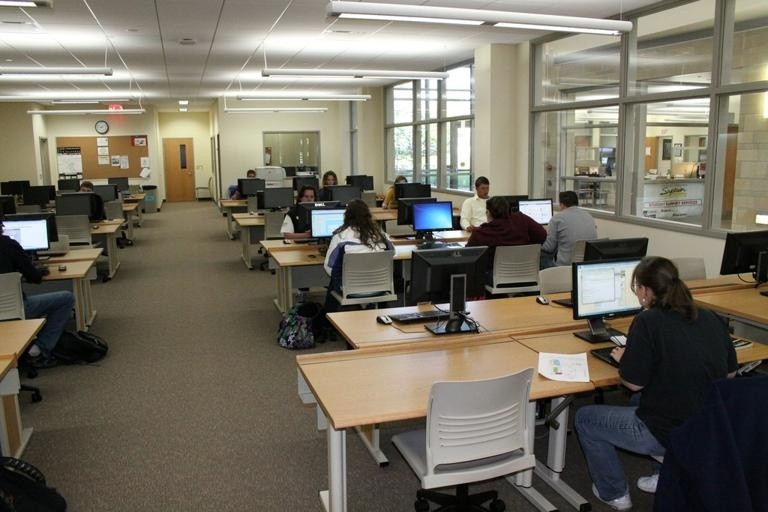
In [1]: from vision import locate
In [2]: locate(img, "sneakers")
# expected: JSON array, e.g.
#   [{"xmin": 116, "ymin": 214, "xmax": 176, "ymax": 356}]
[
  {"xmin": 591, "ymin": 481, "xmax": 635, "ymax": 511},
  {"xmin": 636, "ymin": 473, "xmax": 663, "ymax": 492}
]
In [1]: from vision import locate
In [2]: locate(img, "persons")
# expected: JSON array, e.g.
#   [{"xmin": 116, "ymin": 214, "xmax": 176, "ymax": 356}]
[
  {"xmin": 323, "ymin": 199, "xmax": 397, "ymax": 310},
  {"xmin": 382, "ymin": 175, "xmax": 408, "ymax": 209},
  {"xmin": 463, "ymin": 195, "xmax": 548, "ymax": 299},
  {"xmin": 0, "ymin": 216, "xmax": 76, "ymax": 367},
  {"xmin": 280, "ymin": 184, "xmax": 316, "ymax": 239},
  {"xmin": 77, "ymin": 181, "xmax": 107, "ymax": 219},
  {"xmin": 319, "ymin": 170, "xmax": 338, "ymax": 201},
  {"xmin": 230, "ymin": 170, "xmax": 256, "ymax": 198},
  {"xmin": 540, "ymin": 190, "xmax": 598, "ymax": 268},
  {"xmin": 572, "ymin": 254, "xmax": 738, "ymax": 511},
  {"xmin": 460, "ymin": 175, "xmax": 492, "ymax": 233}
]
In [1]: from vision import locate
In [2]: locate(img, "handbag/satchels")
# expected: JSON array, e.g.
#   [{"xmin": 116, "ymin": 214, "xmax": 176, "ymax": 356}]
[{"xmin": 274, "ymin": 301, "xmax": 316, "ymax": 350}]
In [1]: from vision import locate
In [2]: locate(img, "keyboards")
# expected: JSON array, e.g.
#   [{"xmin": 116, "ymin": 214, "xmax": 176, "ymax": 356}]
[
  {"xmin": 416, "ymin": 243, "xmax": 462, "ymax": 249},
  {"xmin": 389, "ymin": 310, "xmax": 469, "ymax": 322},
  {"xmin": 553, "ymin": 298, "xmax": 572, "ymax": 307},
  {"xmin": 590, "ymin": 346, "xmax": 624, "ymax": 367}
]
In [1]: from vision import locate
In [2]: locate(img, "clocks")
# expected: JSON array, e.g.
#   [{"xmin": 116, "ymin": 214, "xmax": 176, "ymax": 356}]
[{"xmin": 95, "ymin": 120, "xmax": 109, "ymax": 134}]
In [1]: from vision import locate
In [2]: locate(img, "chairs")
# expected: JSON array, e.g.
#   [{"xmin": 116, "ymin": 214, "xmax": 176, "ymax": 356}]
[
  {"xmin": 129, "ymin": 185, "xmax": 140, "ymax": 194},
  {"xmin": 228, "ymin": 185, "xmax": 239, "ymax": 199}
]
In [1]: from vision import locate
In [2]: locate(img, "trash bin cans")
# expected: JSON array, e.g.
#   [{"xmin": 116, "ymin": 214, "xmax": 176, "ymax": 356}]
[{"xmin": 142, "ymin": 185, "xmax": 160, "ymax": 212}]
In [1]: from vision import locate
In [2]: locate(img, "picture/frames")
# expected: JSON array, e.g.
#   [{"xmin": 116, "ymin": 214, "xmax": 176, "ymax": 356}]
[{"xmin": 674, "ymin": 144, "xmax": 682, "ymax": 157}]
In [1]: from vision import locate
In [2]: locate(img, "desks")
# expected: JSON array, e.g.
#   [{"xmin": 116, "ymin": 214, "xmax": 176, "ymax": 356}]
[{"xmin": 0, "ymin": 192, "xmax": 146, "ymax": 461}]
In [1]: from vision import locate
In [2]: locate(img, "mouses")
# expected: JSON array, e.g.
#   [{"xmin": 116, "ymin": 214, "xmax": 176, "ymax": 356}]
[
  {"xmin": 536, "ymin": 296, "xmax": 548, "ymax": 304},
  {"xmin": 377, "ymin": 315, "xmax": 391, "ymax": 324}
]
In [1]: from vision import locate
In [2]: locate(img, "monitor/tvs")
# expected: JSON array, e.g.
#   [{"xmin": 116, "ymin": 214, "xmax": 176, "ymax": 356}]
[
  {"xmin": 396, "ymin": 197, "xmax": 436, "ymax": 225},
  {"xmin": 0, "ymin": 176, "xmax": 129, "ymax": 258},
  {"xmin": 720, "ymin": 229, "xmax": 768, "ymax": 283},
  {"xmin": 517, "ymin": 198, "xmax": 554, "ymax": 225},
  {"xmin": 497, "ymin": 195, "xmax": 529, "ymax": 209},
  {"xmin": 311, "ymin": 210, "xmax": 350, "ymax": 236},
  {"xmin": 299, "ymin": 201, "xmax": 342, "ymax": 229},
  {"xmin": 411, "ymin": 245, "xmax": 488, "ymax": 335},
  {"xmin": 413, "ymin": 201, "xmax": 451, "ymax": 231},
  {"xmin": 237, "ymin": 175, "xmax": 431, "ymax": 209},
  {"xmin": 583, "ymin": 237, "xmax": 648, "ymax": 260},
  {"xmin": 572, "ymin": 258, "xmax": 645, "ymax": 343}
]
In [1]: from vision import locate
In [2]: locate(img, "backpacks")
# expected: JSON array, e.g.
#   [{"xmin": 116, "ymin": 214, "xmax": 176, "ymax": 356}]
[{"xmin": 53, "ymin": 326, "xmax": 111, "ymax": 366}]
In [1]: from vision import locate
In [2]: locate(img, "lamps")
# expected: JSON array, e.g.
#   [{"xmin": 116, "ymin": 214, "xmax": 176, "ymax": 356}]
[
  {"xmin": 237, "ymin": 79, "xmax": 372, "ymax": 101},
  {"xmin": 261, "ymin": 43, "xmax": 449, "ymax": 80},
  {"xmin": 0, "ymin": 0, "xmax": 38, "ymax": 8},
  {"xmin": 223, "ymin": 95, "xmax": 328, "ymax": 114},
  {"xmin": 0, "ymin": 46, "xmax": 113, "ymax": 75},
  {"xmin": 26, "ymin": 98, "xmax": 146, "ymax": 115},
  {"xmin": 324, "ymin": 0, "xmax": 633, "ymax": 36},
  {"xmin": 0, "ymin": 77, "xmax": 133, "ymax": 104}
]
